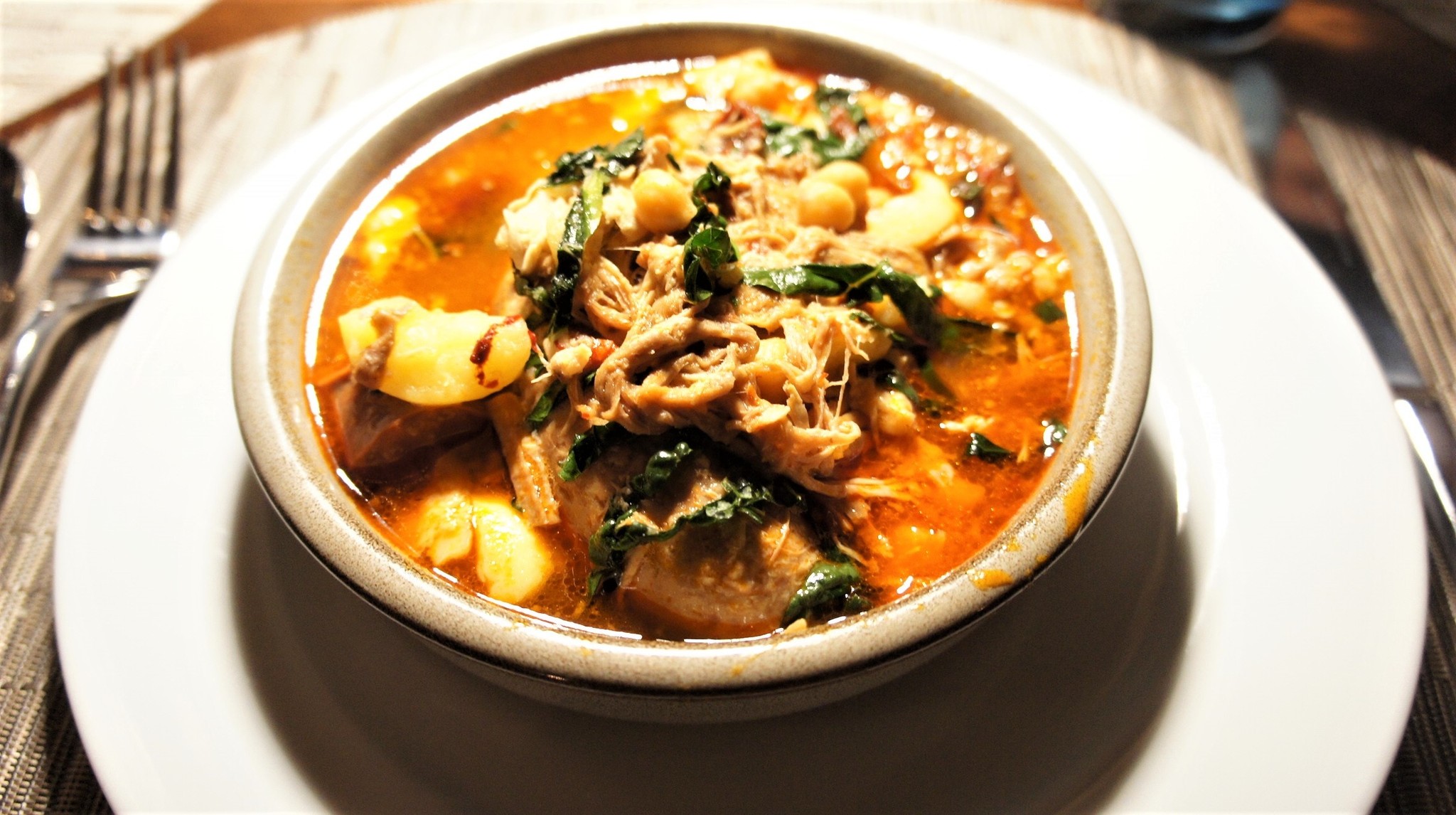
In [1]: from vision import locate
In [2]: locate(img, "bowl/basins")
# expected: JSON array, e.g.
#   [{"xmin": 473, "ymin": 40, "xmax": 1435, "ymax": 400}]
[{"xmin": 228, "ymin": 19, "xmax": 1156, "ymax": 724}]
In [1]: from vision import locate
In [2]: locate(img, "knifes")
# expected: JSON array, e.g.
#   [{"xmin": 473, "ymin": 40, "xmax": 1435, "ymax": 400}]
[{"xmin": 1233, "ymin": 56, "xmax": 1456, "ymax": 558}]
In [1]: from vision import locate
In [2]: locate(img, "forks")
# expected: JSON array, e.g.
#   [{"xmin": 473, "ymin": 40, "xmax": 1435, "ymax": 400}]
[{"xmin": 0, "ymin": 33, "xmax": 194, "ymax": 505}]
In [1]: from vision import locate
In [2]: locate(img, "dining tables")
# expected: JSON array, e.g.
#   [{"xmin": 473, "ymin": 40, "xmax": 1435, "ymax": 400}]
[{"xmin": 0, "ymin": 0, "xmax": 1456, "ymax": 815}]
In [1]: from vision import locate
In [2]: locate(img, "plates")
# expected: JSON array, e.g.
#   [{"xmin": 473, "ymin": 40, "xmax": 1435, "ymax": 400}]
[{"xmin": 57, "ymin": 18, "xmax": 1432, "ymax": 814}]
